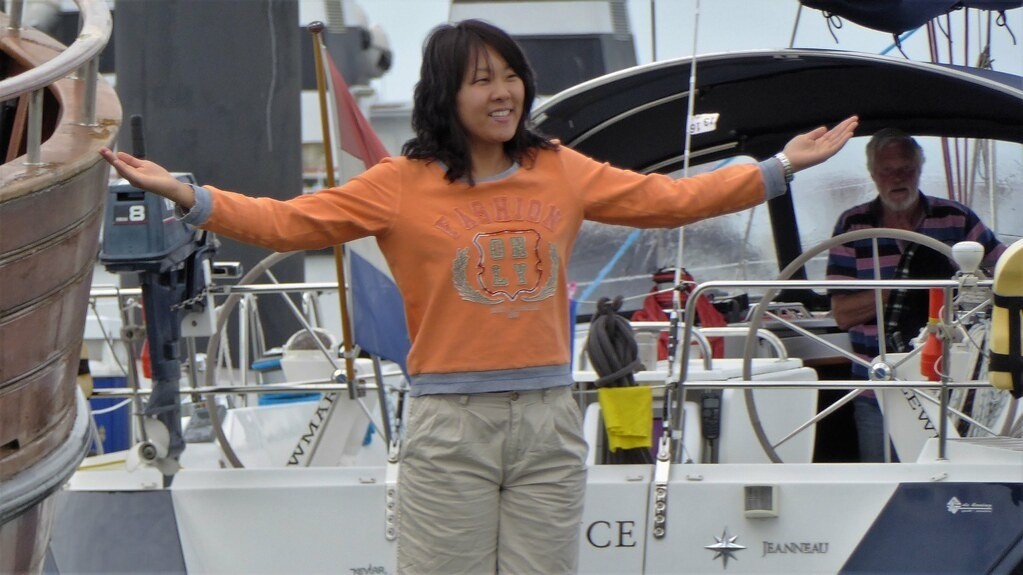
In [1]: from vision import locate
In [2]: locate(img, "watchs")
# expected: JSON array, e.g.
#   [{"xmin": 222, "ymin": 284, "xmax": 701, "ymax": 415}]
[{"xmin": 775, "ymin": 152, "xmax": 794, "ymax": 183}]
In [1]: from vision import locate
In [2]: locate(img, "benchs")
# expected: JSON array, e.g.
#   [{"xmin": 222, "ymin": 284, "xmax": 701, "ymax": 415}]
[{"xmin": 758, "ymin": 317, "xmax": 857, "ymax": 368}]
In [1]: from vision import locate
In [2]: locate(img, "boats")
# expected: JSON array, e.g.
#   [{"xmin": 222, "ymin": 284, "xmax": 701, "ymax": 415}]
[
  {"xmin": 0, "ymin": 1, "xmax": 125, "ymax": 575},
  {"xmin": 0, "ymin": 1, "xmax": 1023, "ymax": 575}
]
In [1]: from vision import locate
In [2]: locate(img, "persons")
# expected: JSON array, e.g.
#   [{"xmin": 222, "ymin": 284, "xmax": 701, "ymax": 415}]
[
  {"xmin": 825, "ymin": 128, "xmax": 1009, "ymax": 463},
  {"xmin": 99, "ymin": 18, "xmax": 859, "ymax": 575}
]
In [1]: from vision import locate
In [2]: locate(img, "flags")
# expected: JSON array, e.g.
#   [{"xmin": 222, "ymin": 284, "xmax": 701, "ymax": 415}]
[{"xmin": 320, "ymin": 40, "xmax": 393, "ymax": 170}]
